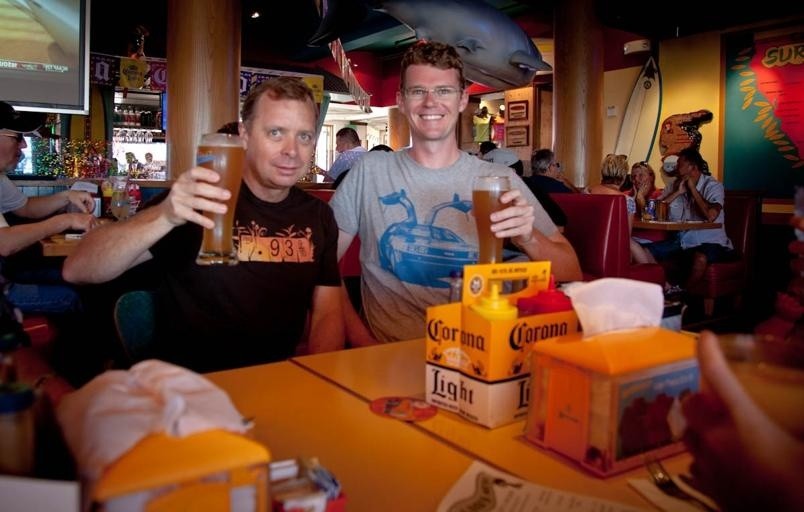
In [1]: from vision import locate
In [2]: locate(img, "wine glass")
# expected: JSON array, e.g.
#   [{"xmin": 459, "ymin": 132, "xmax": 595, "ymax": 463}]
[
  {"xmin": 111, "ymin": 183, "xmax": 131, "ymax": 222},
  {"xmin": 113, "ymin": 128, "xmax": 153, "ymax": 144}
]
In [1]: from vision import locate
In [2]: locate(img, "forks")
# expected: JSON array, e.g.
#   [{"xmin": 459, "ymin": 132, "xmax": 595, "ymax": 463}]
[{"xmin": 643, "ymin": 452, "xmax": 719, "ymax": 512}]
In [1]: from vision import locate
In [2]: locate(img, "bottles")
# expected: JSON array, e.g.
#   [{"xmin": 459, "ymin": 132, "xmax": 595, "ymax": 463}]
[
  {"xmin": 0, "ymin": 381, "xmax": 40, "ymax": 477},
  {"xmin": 448, "ymin": 270, "xmax": 464, "ymax": 303},
  {"xmin": 113, "ymin": 105, "xmax": 162, "ymax": 128}
]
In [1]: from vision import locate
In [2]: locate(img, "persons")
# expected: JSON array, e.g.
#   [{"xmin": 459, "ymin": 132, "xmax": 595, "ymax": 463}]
[
  {"xmin": 681, "ymin": 330, "xmax": 804, "ymax": 512},
  {"xmin": 479, "ymin": 142, "xmax": 523, "ymax": 176},
  {"xmin": 144, "ymin": 152, "xmax": 161, "ymax": 171},
  {"xmin": 62, "ymin": 75, "xmax": 343, "ymax": 372},
  {"xmin": 520, "ymin": 149, "xmax": 578, "ymax": 236},
  {"xmin": 0, "ymin": 101, "xmax": 103, "ymax": 313},
  {"xmin": 584, "ymin": 149, "xmax": 737, "ymax": 332},
  {"xmin": 329, "ymin": 43, "xmax": 583, "ymax": 343},
  {"xmin": 324, "ymin": 127, "xmax": 367, "ymax": 181}
]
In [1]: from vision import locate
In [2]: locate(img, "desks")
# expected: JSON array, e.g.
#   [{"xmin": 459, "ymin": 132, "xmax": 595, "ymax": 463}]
[{"xmin": 200, "ymin": 327, "xmax": 803, "ymax": 511}]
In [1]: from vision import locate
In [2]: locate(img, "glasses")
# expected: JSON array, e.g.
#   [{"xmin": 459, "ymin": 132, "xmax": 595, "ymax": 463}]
[
  {"xmin": 400, "ymin": 86, "xmax": 460, "ymax": 100},
  {"xmin": 0, "ymin": 133, "xmax": 23, "ymax": 142},
  {"xmin": 533, "ymin": 167, "xmax": 547, "ymax": 174},
  {"xmin": 402, "ymin": 85, "xmax": 460, "ymax": 104},
  {"xmin": 606, "ymin": 153, "xmax": 626, "ymax": 159}
]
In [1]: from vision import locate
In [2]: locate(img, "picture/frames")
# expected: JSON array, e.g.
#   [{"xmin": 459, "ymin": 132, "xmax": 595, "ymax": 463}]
[
  {"xmin": 507, "ymin": 100, "xmax": 529, "ymax": 121},
  {"xmin": 506, "ymin": 124, "xmax": 529, "ymax": 148}
]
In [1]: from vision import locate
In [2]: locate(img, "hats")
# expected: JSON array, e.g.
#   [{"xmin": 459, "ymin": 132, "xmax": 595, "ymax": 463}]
[
  {"xmin": 0, "ymin": 100, "xmax": 49, "ymax": 134},
  {"xmin": 483, "ymin": 149, "xmax": 520, "ymax": 167}
]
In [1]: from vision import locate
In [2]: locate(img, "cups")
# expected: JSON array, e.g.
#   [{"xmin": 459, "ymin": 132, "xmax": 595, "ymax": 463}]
[
  {"xmin": 472, "ymin": 174, "xmax": 515, "ymax": 265},
  {"xmin": 195, "ymin": 132, "xmax": 247, "ymax": 267},
  {"xmin": 695, "ymin": 332, "xmax": 803, "ymax": 446}
]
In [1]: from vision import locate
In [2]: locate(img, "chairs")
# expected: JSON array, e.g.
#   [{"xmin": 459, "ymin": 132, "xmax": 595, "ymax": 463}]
[
  {"xmin": 685, "ymin": 188, "xmax": 767, "ymax": 325},
  {"xmin": 545, "ymin": 194, "xmax": 665, "ymax": 282}
]
